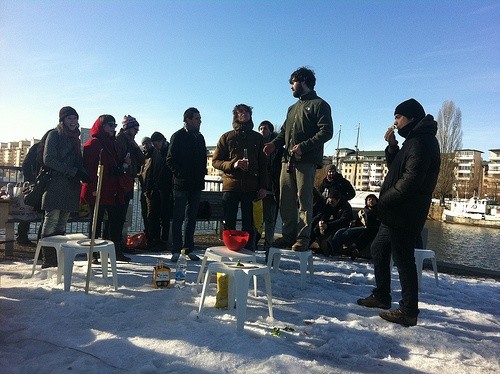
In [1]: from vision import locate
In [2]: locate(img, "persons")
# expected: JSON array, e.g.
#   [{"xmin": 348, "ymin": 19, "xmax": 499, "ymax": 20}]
[
  {"xmin": 263, "ymin": 68, "xmax": 334, "ymax": 251},
  {"xmin": 140, "ymin": 132, "xmax": 174, "ymax": 252},
  {"xmin": 296, "ymin": 165, "xmax": 379, "ymax": 262},
  {"xmin": 118, "ymin": 115, "xmax": 144, "ymax": 254},
  {"xmin": 356, "ymin": 97, "xmax": 441, "ymax": 330},
  {"xmin": 16, "ymin": 130, "xmax": 56, "ymax": 247},
  {"xmin": 212, "ymin": 104, "xmax": 264, "ymax": 253},
  {"xmin": 165, "ymin": 108, "xmax": 208, "ymax": 263},
  {"xmin": 80, "ymin": 115, "xmax": 132, "ymax": 264},
  {"xmin": 26, "ymin": 211, "xmax": 43, "ymax": 265},
  {"xmin": 40, "ymin": 106, "xmax": 89, "ymax": 272},
  {"xmin": 255, "ymin": 120, "xmax": 286, "ymax": 239}
]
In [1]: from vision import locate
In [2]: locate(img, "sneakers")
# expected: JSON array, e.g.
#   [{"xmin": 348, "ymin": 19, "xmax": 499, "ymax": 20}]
[
  {"xmin": 379, "ymin": 307, "xmax": 417, "ymax": 327},
  {"xmin": 357, "ymin": 294, "xmax": 391, "ymax": 310}
]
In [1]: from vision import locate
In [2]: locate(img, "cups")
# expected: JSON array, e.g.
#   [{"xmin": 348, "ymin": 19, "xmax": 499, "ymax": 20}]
[
  {"xmin": 1, "ymin": 186, "xmax": 7, "ymax": 196},
  {"xmin": 319, "ymin": 221, "xmax": 324, "ymax": 234},
  {"xmin": 7, "ymin": 183, "xmax": 14, "ymax": 198},
  {"xmin": 324, "ymin": 188, "xmax": 328, "ymax": 199},
  {"xmin": 12, "ymin": 187, "xmax": 21, "ymax": 197}
]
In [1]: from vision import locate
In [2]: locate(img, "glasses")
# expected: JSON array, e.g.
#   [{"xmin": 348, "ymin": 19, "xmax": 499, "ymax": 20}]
[{"xmin": 104, "ymin": 123, "xmax": 117, "ymax": 127}]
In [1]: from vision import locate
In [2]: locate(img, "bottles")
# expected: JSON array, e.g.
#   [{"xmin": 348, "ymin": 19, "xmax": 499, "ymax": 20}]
[
  {"xmin": 121, "ymin": 153, "xmax": 130, "ymax": 175},
  {"xmin": 174, "ymin": 249, "xmax": 188, "ymax": 288},
  {"xmin": 152, "ymin": 260, "xmax": 171, "ymax": 289},
  {"xmin": 243, "ymin": 148, "xmax": 248, "ymax": 171}
]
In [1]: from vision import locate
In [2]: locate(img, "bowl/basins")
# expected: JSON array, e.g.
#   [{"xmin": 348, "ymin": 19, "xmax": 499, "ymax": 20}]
[{"xmin": 223, "ymin": 230, "xmax": 249, "ymax": 251}]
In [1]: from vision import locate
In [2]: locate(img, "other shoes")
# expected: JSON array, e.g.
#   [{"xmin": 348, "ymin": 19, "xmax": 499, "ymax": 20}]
[
  {"xmin": 272, "ymin": 237, "xmax": 297, "ymax": 248},
  {"xmin": 92, "ymin": 257, "xmax": 98, "ymax": 264},
  {"xmin": 116, "ymin": 254, "xmax": 132, "ymax": 261},
  {"xmin": 317, "ymin": 236, "xmax": 332, "ymax": 255},
  {"xmin": 188, "ymin": 252, "xmax": 200, "ymax": 261},
  {"xmin": 292, "ymin": 241, "xmax": 309, "ymax": 252},
  {"xmin": 17, "ymin": 240, "xmax": 37, "ymax": 248},
  {"xmin": 171, "ymin": 253, "xmax": 180, "ymax": 261}
]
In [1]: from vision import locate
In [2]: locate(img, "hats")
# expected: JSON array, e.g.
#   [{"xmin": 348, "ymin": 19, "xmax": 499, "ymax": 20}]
[
  {"xmin": 59, "ymin": 107, "xmax": 78, "ymax": 122},
  {"xmin": 122, "ymin": 115, "xmax": 140, "ymax": 129},
  {"xmin": 142, "ymin": 137, "xmax": 151, "ymax": 146},
  {"xmin": 394, "ymin": 98, "xmax": 426, "ymax": 121}
]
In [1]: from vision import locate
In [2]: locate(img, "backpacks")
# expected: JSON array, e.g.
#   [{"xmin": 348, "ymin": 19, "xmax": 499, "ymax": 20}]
[{"xmin": 23, "ymin": 129, "xmax": 55, "ymax": 205}]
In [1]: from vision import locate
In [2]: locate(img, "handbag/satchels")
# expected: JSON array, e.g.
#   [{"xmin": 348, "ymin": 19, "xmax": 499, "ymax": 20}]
[{"xmin": 253, "ymin": 198, "xmax": 263, "ymax": 234}]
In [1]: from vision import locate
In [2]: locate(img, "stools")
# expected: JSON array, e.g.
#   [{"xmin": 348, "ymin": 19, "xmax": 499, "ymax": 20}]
[
  {"xmin": 197, "ymin": 262, "xmax": 273, "ymax": 334},
  {"xmin": 32, "ymin": 233, "xmax": 117, "ymax": 291},
  {"xmin": 267, "ymin": 246, "xmax": 314, "ymax": 290},
  {"xmin": 390, "ymin": 248, "xmax": 438, "ymax": 292},
  {"xmin": 196, "ymin": 246, "xmax": 258, "ymax": 298}
]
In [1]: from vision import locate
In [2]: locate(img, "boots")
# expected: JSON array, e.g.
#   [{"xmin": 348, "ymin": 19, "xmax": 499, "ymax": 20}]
[
  {"xmin": 41, "ymin": 232, "xmax": 58, "ymax": 269},
  {"xmin": 56, "ymin": 231, "xmax": 66, "ymax": 235}
]
